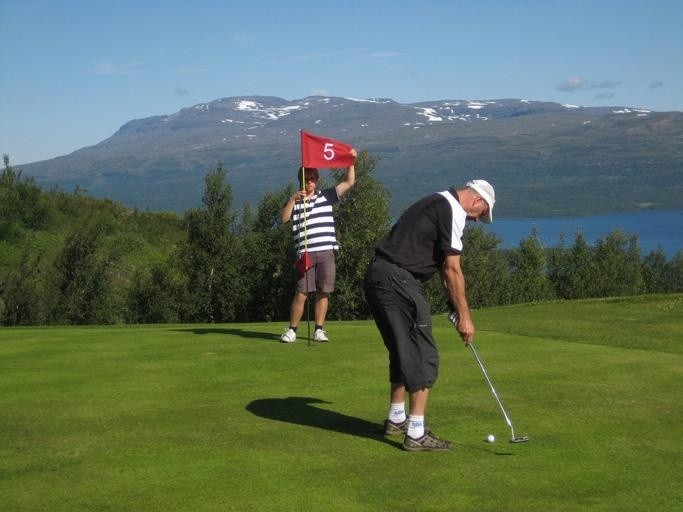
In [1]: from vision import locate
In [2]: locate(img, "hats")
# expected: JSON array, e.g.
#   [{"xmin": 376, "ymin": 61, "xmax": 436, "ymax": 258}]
[{"xmin": 466, "ymin": 180, "xmax": 496, "ymax": 224}]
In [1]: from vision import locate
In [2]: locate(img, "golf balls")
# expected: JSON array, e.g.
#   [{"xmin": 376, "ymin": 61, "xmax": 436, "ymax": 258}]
[{"xmin": 487, "ymin": 435, "xmax": 495, "ymax": 442}]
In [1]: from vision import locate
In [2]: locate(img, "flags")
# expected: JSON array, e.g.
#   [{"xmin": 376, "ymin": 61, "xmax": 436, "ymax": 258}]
[{"xmin": 300, "ymin": 129, "xmax": 353, "ymax": 169}]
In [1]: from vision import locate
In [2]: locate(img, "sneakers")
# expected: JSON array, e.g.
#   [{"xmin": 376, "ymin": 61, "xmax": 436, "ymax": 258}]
[
  {"xmin": 383, "ymin": 415, "xmax": 431, "ymax": 436},
  {"xmin": 280, "ymin": 328, "xmax": 296, "ymax": 343},
  {"xmin": 313, "ymin": 329, "xmax": 328, "ymax": 342},
  {"xmin": 401, "ymin": 430, "xmax": 453, "ymax": 452}
]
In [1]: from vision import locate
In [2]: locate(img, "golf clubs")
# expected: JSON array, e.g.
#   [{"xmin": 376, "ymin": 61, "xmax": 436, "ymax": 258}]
[{"xmin": 449, "ymin": 310, "xmax": 530, "ymax": 443}]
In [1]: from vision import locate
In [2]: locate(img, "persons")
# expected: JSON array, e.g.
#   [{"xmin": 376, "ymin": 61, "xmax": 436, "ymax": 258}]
[
  {"xmin": 280, "ymin": 149, "xmax": 357, "ymax": 343},
  {"xmin": 363, "ymin": 178, "xmax": 496, "ymax": 452}
]
